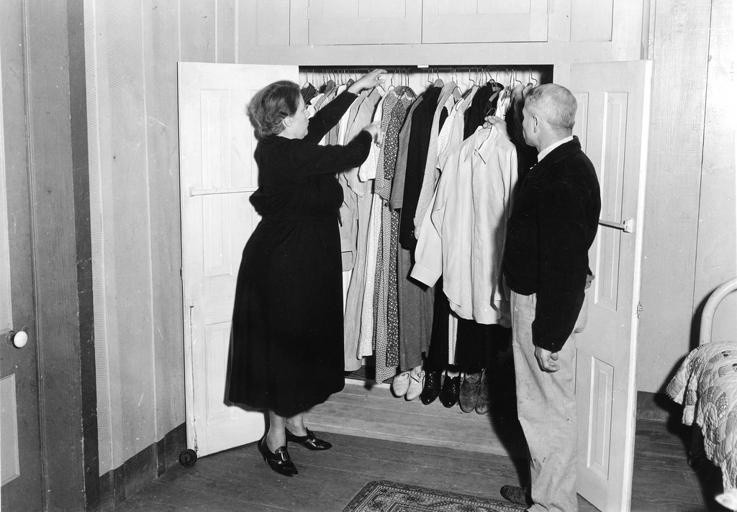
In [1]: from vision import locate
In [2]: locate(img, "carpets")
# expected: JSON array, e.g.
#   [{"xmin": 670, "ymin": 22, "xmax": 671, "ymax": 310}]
[{"xmin": 339, "ymin": 479, "xmax": 536, "ymax": 512}]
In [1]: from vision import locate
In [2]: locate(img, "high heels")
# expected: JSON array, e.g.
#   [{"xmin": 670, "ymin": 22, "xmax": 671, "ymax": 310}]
[
  {"xmin": 285, "ymin": 427, "xmax": 331, "ymax": 450},
  {"xmin": 261, "ymin": 435, "xmax": 298, "ymax": 477}
]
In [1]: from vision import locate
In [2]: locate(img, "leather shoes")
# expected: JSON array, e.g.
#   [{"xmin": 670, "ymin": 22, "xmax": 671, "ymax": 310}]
[
  {"xmin": 392, "ymin": 368, "xmax": 493, "ymax": 414},
  {"xmin": 501, "ymin": 485, "xmax": 532, "ymax": 507}
]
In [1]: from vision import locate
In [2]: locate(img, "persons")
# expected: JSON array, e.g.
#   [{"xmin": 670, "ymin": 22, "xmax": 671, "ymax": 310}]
[
  {"xmin": 499, "ymin": 83, "xmax": 604, "ymax": 512},
  {"xmin": 221, "ymin": 67, "xmax": 389, "ymax": 477}
]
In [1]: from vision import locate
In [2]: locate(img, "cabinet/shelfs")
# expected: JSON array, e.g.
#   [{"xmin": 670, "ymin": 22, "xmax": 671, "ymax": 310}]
[{"xmin": 176, "ymin": 0, "xmax": 653, "ymax": 512}]
